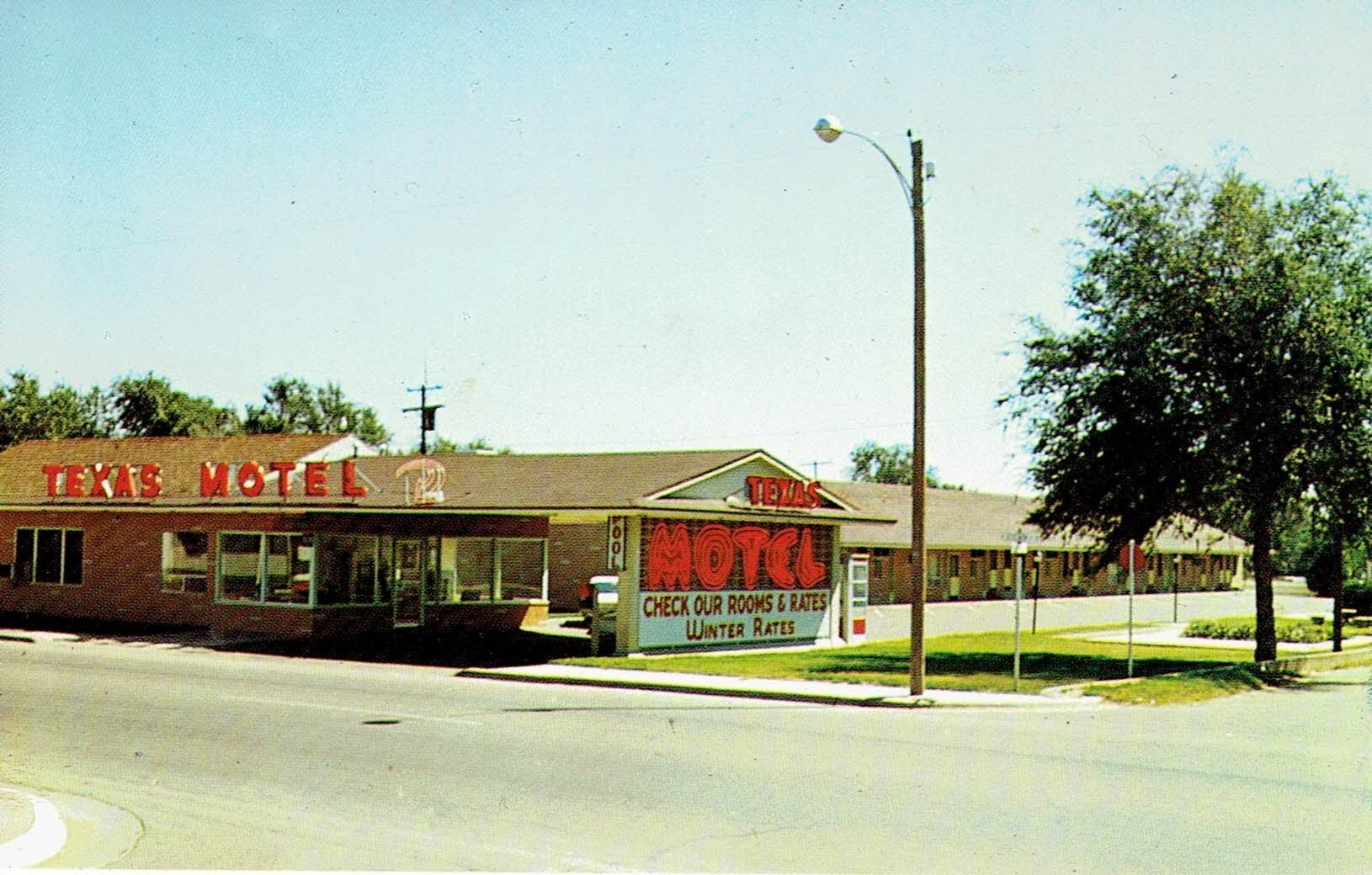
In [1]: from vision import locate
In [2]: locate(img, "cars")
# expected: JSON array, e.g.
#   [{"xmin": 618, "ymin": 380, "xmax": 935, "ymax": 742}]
[{"xmin": 578, "ymin": 575, "xmax": 619, "ymax": 621}]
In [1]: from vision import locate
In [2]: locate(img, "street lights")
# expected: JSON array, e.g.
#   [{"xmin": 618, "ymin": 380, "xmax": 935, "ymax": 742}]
[{"xmin": 815, "ymin": 111, "xmax": 927, "ymax": 695}]
